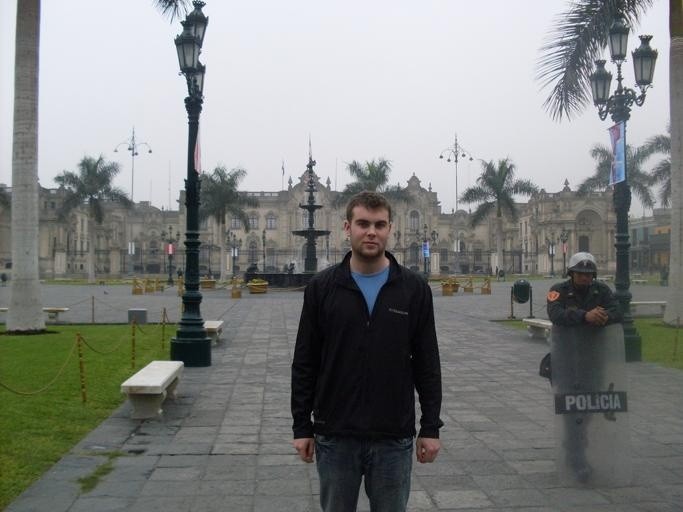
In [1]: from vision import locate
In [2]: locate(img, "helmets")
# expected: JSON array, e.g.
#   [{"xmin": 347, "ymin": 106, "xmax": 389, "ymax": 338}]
[{"xmin": 567, "ymin": 252, "xmax": 597, "ymax": 274}]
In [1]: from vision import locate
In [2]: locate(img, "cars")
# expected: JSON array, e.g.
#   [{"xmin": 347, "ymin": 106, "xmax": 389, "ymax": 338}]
[{"xmin": 199, "ymin": 264, "xmax": 209, "ymax": 277}]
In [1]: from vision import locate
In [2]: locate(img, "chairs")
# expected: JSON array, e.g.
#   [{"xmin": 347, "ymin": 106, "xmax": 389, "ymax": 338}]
[
  {"xmin": 521, "ymin": 297, "xmax": 668, "ymax": 350},
  {"xmin": 512, "ymin": 271, "xmax": 647, "ymax": 286}
]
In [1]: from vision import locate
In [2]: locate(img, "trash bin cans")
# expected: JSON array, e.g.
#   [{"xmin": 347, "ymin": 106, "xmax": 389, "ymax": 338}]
[{"xmin": 509, "ymin": 279, "xmax": 535, "ymax": 319}]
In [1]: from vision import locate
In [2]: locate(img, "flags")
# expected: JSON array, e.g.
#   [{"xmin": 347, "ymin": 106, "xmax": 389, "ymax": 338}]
[
  {"xmin": 421, "ymin": 239, "xmax": 430, "ymax": 258},
  {"xmin": 606, "ymin": 117, "xmax": 627, "ymax": 186}
]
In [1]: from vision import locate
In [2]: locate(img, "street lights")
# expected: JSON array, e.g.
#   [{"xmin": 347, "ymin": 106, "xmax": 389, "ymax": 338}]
[
  {"xmin": 545, "ymin": 232, "xmax": 569, "ymax": 278},
  {"xmin": 262, "ymin": 230, "xmax": 266, "ymax": 270},
  {"xmin": 113, "ymin": 124, "xmax": 152, "ymax": 276},
  {"xmin": 416, "ymin": 224, "xmax": 438, "ymax": 272},
  {"xmin": 227, "ymin": 234, "xmax": 242, "ymax": 279},
  {"xmin": 589, "ymin": 12, "xmax": 658, "ymax": 361},
  {"xmin": 439, "ymin": 131, "xmax": 473, "ymax": 211},
  {"xmin": 161, "ymin": 225, "xmax": 181, "ymax": 285},
  {"xmin": 171, "ymin": 0, "xmax": 212, "ymax": 367}
]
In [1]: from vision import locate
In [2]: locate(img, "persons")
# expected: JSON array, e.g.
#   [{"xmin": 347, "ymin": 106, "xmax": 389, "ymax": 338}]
[
  {"xmin": 545, "ymin": 250, "xmax": 625, "ymax": 479},
  {"xmin": 288, "ymin": 188, "xmax": 443, "ymax": 512}
]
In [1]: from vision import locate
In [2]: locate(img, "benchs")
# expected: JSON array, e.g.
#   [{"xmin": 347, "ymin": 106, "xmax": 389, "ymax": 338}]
[{"xmin": 0, "ymin": 305, "xmax": 225, "ymax": 421}]
